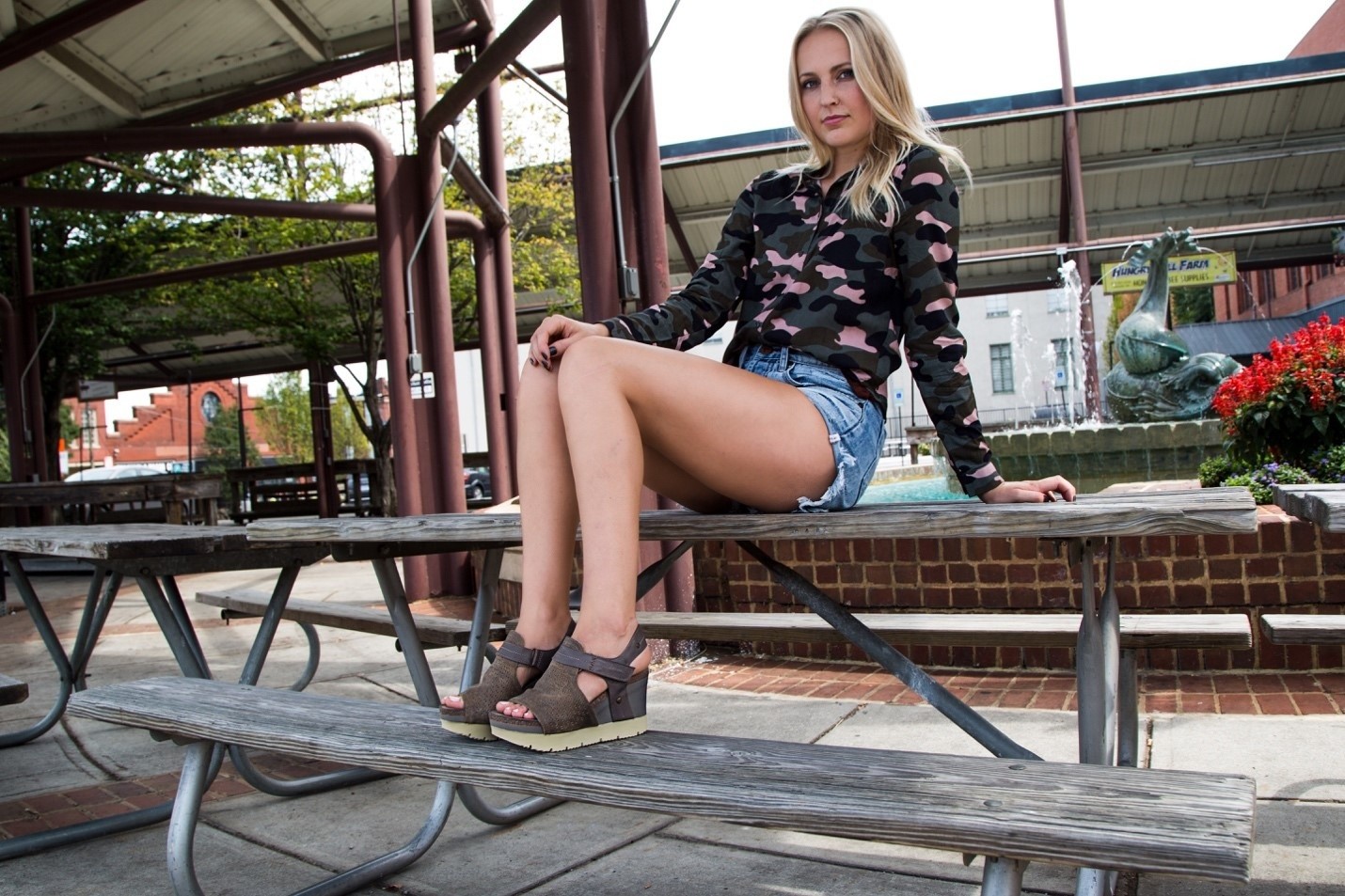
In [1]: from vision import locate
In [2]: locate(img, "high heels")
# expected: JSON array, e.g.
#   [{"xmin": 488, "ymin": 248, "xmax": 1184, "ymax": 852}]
[
  {"xmin": 439, "ymin": 619, "xmax": 577, "ymax": 742},
  {"xmin": 488, "ymin": 623, "xmax": 651, "ymax": 751}
]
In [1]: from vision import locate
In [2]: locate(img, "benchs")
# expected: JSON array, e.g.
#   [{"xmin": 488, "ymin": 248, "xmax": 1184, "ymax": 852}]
[{"xmin": 0, "ymin": 590, "xmax": 1344, "ymax": 896}]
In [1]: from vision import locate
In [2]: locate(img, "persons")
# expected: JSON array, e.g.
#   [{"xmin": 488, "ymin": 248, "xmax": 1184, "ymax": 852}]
[{"xmin": 426, "ymin": 5, "xmax": 1077, "ymax": 750}]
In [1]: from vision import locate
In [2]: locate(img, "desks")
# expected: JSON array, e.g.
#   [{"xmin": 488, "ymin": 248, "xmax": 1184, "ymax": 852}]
[
  {"xmin": 0, "ymin": 521, "xmax": 504, "ymax": 857},
  {"xmin": 165, "ymin": 487, "xmax": 1260, "ymax": 895}
]
